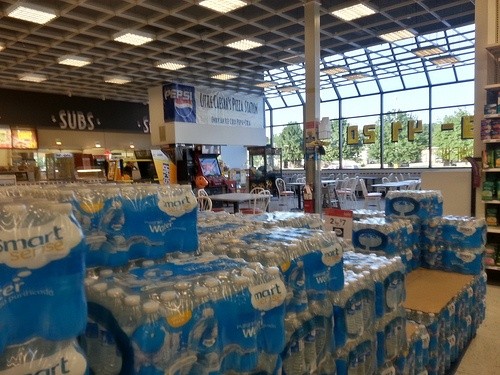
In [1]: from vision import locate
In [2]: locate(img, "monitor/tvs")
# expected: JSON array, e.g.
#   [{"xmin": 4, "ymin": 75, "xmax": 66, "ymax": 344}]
[{"xmin": 199, "ymin": 158, "xmax": 220, "ymax": 176}]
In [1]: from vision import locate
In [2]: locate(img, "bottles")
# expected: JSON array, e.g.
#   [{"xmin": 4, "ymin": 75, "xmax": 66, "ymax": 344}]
[{"xmin": 0, "ymin": 183, "xmax": 487, "ymax": 375}]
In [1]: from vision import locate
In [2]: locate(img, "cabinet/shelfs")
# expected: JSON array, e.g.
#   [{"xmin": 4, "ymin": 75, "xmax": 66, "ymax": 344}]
[{"xmin": 480, "ymin": 84, "xmax": 500, "ymax": 270}]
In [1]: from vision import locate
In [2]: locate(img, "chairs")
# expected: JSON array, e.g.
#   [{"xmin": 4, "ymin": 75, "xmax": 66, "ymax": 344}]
[
  {"xmin": 240, "ymin": 187, "xmax": 270, "ymax": 214},
  {"xmin": 275, "ymin": 173, "xmax": 423, "ymax": 211},
  {"xmin": 196, "ymin": 189, "xmax": 225, "ymax": 212}
]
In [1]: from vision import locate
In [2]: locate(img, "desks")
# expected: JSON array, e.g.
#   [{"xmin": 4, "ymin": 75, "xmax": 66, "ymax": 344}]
[
  {"xmin": 286, "ymin": 180, "xmax": 337, "ymax": 210},
  {"xmin": 209, "ymin": 192, "xmax": 273, "ymax": 214},
  {"xmin": 371, "ymin": 180, "xmax": 421, "ymax": 195},
  {"xmin": 358, "ymin": 177, "xmax": 378, "ymax": 193}
]
